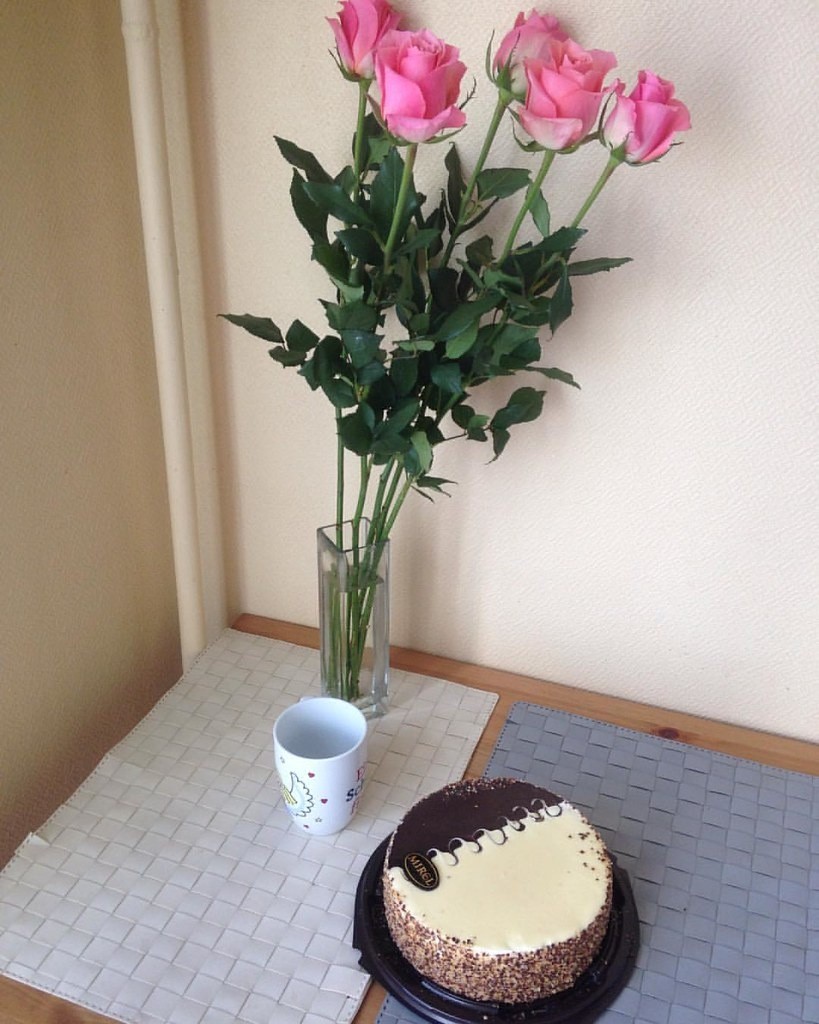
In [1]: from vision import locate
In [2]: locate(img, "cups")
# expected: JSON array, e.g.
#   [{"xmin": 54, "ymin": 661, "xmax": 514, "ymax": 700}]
[{"xmin": 272, "ymin": 696, "xmax": 370, "ymax": 835}]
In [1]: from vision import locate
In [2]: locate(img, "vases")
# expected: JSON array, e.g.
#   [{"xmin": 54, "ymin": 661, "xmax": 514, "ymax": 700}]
[{"xmin": 316, "ymin": 518, "xmax": 395, "ymax": 718}]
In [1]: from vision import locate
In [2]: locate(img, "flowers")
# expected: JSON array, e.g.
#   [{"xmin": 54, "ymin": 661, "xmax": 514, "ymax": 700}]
[{"xmin": 211, "ymin": 2, "xmax": 693, "ymax": 698}]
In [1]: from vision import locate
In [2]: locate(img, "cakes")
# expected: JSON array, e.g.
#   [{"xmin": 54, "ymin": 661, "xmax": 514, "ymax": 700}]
[{"xmin": 381, "ymin": 778, "xmax": 613, "ymax": 1004}]
